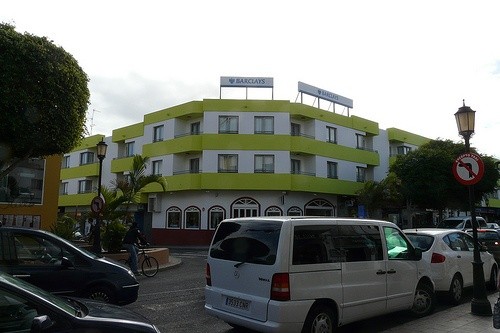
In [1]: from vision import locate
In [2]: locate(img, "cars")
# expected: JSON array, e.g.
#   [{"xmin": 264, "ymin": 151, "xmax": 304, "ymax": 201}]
[
  {"xmin": 388, "ymin": 228, "xmax": 500, "ymax": 306},
  {"xmin": 0, "ymin": 270, "xmax": 162, "ymax": 333},
  {"xmin": 435, "ymin": 216, "xmax": 500, "ymax": 259},
  {"xmin": 0, "ymin": 227, "xmax": 140, "ymax": 306}
]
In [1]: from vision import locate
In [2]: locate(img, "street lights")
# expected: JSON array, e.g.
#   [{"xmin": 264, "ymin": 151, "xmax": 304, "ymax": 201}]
[
  {"xmin": 93, "ymin": 137, "xmax": 108, "ymax": 254},
  {"xmin": 454, "ymin": 99, "xmax": 493, "ymax": 316}
]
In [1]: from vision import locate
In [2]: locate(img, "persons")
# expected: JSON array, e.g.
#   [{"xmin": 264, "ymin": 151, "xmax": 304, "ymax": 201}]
[{"xmin": 81, "ymin": 217, "xmax": 150, "ymax": 274}]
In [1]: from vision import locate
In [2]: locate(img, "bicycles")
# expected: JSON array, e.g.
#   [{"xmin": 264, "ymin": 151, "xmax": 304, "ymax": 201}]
[{"xmin": 116, "ymin": 244, "xmax": 159, "ymax": 278}]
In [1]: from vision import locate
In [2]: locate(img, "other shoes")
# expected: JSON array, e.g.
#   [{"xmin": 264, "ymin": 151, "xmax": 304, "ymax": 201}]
[{"xmin": 133, "ymin": 271, "xmax": 143, "ymax": 276}]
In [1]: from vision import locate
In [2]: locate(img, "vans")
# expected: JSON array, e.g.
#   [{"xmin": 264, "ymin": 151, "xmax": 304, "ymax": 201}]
[{"xmin": 204, "ymin": 216, "xmax": 436, "ymax": 333}]
